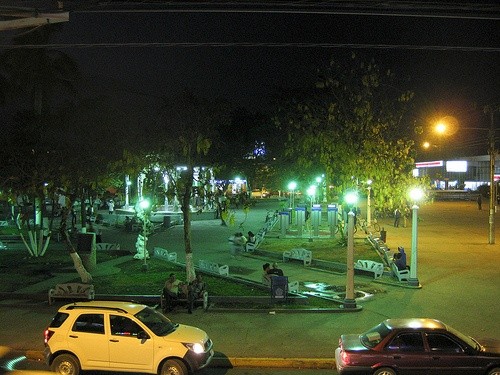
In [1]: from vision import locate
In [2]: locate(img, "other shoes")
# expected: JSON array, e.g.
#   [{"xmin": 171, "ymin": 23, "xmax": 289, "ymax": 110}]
[
  {"xmin": 164, "ymin": 309, "xmax": 169, "ymax": 313},
  {"xmin": 188, "ymin": 309, "xmax": 192, "ymax": 314}
]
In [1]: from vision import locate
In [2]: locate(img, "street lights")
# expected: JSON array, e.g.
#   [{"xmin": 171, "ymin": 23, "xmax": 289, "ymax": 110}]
[
  {"xmin": 344, "ymin": 193, "xmax": 358, "ymax": 300},
  {"xmin": 289, "ymin": 182, "xmax": 296, "ymax": 210},
  {"xmin": 436, "ymin": 124, "xmax": 495, "ymax": 245},
  {"xmin": 140, "ymin": 201, "xmax": 149, "ymax": 271},
  {"xmin": 366, "ymin": 179, "xmax": 373, "ymax": 227},
  {"xmin": 408, "ymin": 187, "xmax": 424, "ymax": 285}
]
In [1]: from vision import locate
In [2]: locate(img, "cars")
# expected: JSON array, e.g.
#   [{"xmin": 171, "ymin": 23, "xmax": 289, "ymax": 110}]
[{"xmin": 335, "ymin": 318, "xmax": 500, "ymax": 375}]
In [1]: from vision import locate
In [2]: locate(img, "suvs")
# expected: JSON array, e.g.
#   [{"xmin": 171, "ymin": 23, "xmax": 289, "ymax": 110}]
[{"xmin": 44, "ymin": 300, "xmax": 216, "ymax": 375}]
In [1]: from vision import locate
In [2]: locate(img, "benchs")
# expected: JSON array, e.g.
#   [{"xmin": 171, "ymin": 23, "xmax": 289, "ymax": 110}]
[
  {"xmin": 0, "ymin": 220, "xmax": 8, "ymax": 227},
  {"xmin": 369, "ymin": 232, "xmax": 380, "ymax": 246},
  {"xmin": 262, "ymin": 274, "xmax": 299, "ymax": 294},
  {"xmin": 160, "ymin": 288, "xmax": 209, "ymax": 311},
  {"xmin": 90, "ymin": 244, "xmax": 120, "ymax": 250},
  {"xmin": 375, "ymin": 240, "xmax": 389, "ymax": 254},
  {"xmin": 353, "ymin": 259, "xmax": 384, "ymax": 279},
  {"xmin": 49, "ymin": 282, "xmax": 95, "ymax": 306},
  {"xmin": 102, "ymin": 217, "xmax": 117, "ymax": 228},
  {"xmin": 152, "ymin": 246, "xmax": 177, "ymax": 263},
  {"xmin": 229, "ymin": 230, "xmax": 266, "ymax": 252},
  {"xmin": 382, "ymin": 249, "xmax": 396, "ymax": 267},
  {"xmin": 390, "ymin": 261, "xmax": 410, "ymax": 282},
  {"xmin": 131, "ymin": 223, "xmax": 150, "ymax": 233},
  {"xmin": 198, "ymin": 258, "xmax": 229, "ymax": 276},
  {"xmin": 282, "ymin": 248, "xmax": 313, "ymax": 267}
]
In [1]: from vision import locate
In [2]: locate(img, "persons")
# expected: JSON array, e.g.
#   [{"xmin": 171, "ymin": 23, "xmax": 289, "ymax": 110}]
[
  {"xmin": 108, "ymin": 200, "xmax": 114, "ymax": 214},
  {"xmin": 263, "ymin": 262, "xmax": 284, "ymax": 284},
  {"xmin": 188, "ymin": 274, "xmax": 207, "ymax": 314},
  {"xmin": 216, "ymin": 203, "xmax": 222, "ymax": 219},
  {"xmin": 402, "ymin": 205, "xmax": 410, "ymax": 228},
  {"xmin": 395, "ymin": 260, "xmax": 406, "ymax": 271},
  {"xmin": 394, "ymin": 207, "xmax": 402, "ymax": 227},
  {"xmin": 477, "ymin": 195, "xmax": 482, "ymax": 210},
  {"xmin": 234, "ymin": 231, "xmax": 255, "ymax": 249},
  {"xmin": 87, "ymin": 207, "xmax": 91, "ymax": 223},
  {"xmin": 163, "ymin": 273, "xmax": 184, "ymax": 315},
  {"xmin": 60, "ymin": 206, "xmax": 65, "ymax": 225},
  {"xmin": 71, "ymin": 206, "xmax": 77, "ymax": 225}
]
